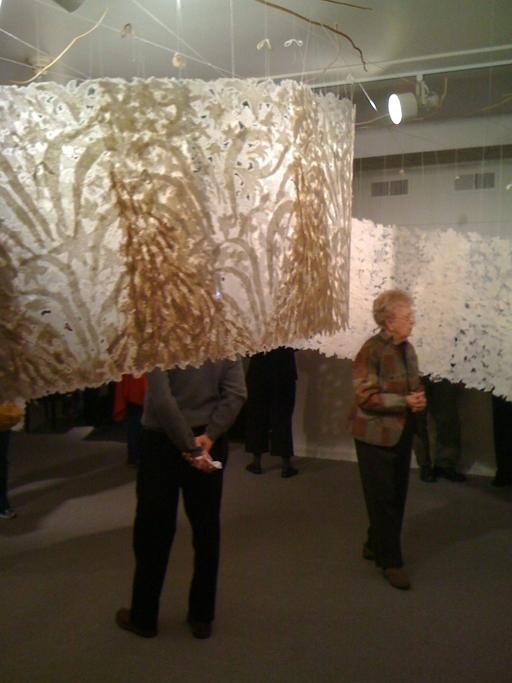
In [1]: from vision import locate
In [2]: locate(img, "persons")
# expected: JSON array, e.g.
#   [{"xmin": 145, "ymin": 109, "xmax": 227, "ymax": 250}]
[
  {"xmin": 346, "ymin": 290, "xmax": 428, "ymax": 591},
  {"xmin": 115, "ymin": 354, "xmax": 248, "ymax": 639},
  {"xmin": 410, "ymin": 374, "xmax": 465, "ymax": 483},
  {"xmin": 490, "ymin": 391, "xmax": 512, "ymax": 487},
  {"xmin": 0, "ymin": 346, "xmax": 300, "ymax": 520}
]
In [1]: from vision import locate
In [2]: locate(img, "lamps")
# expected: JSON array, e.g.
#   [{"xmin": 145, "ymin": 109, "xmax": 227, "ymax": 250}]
[{"xmin": 387, "ymin": 74, "xmax": 440, "ymax": 125}]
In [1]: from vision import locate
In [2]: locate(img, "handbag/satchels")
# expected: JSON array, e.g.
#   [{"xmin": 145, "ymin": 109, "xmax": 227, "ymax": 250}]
[{"xmin": 0, "ymin": 401, "xmax": 21, "ymax": 433}]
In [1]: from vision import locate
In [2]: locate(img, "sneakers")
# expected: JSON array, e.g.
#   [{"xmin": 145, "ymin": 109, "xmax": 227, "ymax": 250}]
[
  {"xmin": 117, "ymin": 608, "xmax": 158, "ymax": 638},
  {"xmin": 282, "ymin": 467, "xmax": 298, "ymax": 478},
  {"xmin": 246, "ymin": 463, "xmax": 262, "ymax": 474},
  {"xmin": 434, "ymin": 467, "xmax": 463, "ymax": 482},
  {"xmin": 362, "ymin": 544, "xmax": 376, "ymax": 560},
  {"xmin": 382, "ymin": 566, "xmax": 409, "ymax": 589},
  {"xmin": 0, "ymin": 508, "xmax": 16, "ymax": 520},
  {"xmin": 419, "ymin": 468, "xmax": 436, "ymax": 482},
  {"xmin": 492, "ymin": 476, "xmax": 508, "ymax": 486},
  {"xmin": 186, "ymin": 612, "xmax": 211, "ymax": 640}
]
